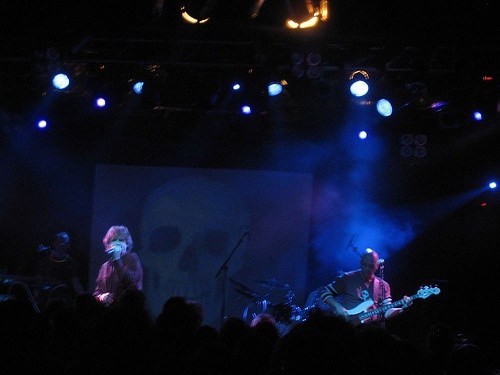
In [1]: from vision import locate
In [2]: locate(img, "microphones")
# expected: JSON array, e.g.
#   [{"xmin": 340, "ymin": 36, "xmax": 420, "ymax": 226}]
[
  {"xmin": 106, "ymin": 246, "xmax": 122, "ymax": 254},
  {"xmin": 347, "ymin": 232, "xmax": 356, "ymax": 249},
  {"xmin": 377, "ymin": 258, "xmax": 385, "ymax": 264}
]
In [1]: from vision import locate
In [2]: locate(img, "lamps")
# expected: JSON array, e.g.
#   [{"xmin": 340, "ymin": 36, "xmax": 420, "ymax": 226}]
[{"xmin": 230, "ymin": 48, "xmax": 397, "ymax": 118}]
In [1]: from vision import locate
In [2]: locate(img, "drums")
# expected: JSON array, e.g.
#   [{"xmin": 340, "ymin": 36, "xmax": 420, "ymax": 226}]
[{"xmin": 272, "ymin": 303, "xmax": 301, "ymax": 325}]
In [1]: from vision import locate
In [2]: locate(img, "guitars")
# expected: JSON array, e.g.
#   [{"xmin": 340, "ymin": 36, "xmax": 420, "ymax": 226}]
[{"xmin": 333, "ymin": 282, "xmax": 440, "ymax": 325}]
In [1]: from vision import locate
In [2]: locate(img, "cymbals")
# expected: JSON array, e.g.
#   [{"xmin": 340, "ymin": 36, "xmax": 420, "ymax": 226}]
[{"xmin": 255, "ymin": 279, "xmax": 290, "ymax": 291}]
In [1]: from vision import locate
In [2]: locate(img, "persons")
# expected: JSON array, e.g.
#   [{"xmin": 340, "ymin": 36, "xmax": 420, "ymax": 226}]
[
  {"xmin": 0, "ymin": 277, "xmax": 500, "ymax": 375},
  {"xmin": 92, "ymin": 225, "xmax": 142, "ymax": 308},
  {"xmin": 20, "ymin": 231, "xmax": 86, "ymax": 308},
  {"xmin": 318, "ymin": 248, "xmax": 392, "ymax": 330}
]
